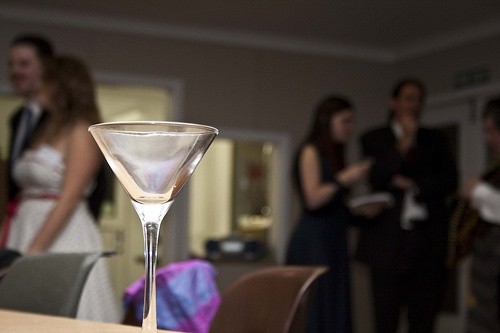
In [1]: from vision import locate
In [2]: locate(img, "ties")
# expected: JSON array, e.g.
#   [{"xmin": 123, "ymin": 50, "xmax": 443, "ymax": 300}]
[
  {"xmin": 16, "ymin": 110, "xmax": 34, "ymax": 152},
  {"xmin": 397, "ymin": 128, "xmax": 413, "ymax": 155}
]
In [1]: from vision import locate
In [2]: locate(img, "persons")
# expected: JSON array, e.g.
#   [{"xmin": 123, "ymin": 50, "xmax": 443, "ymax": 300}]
[
  {"xmin": 4, "ymin": 34, "xmax": 65, "ymax": 204},
  {"xmin": 457, "ymin": 96, "xmax": 500, "ymax": 333},
  {"xmin": 283, "ymin": 94, "xmax": 370, "ymax": 332},
  {"xmin": 7, "ymin": 56, "xmax": 126, "ymax": 326},
  {"xmin": 358, "ymin": 78, "xmax": 455, "ymax": 332}
]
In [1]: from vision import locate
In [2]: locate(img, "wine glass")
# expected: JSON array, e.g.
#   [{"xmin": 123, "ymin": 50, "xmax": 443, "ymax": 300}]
[{"xmin": 88, "ymin": 120, "xmax": 218, "ymax": 333}]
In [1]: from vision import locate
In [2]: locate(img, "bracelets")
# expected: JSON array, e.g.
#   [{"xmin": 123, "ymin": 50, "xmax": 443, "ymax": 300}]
[{"xmin": 325, "ymin": 176, "xmax": 347, "ymax": 193}]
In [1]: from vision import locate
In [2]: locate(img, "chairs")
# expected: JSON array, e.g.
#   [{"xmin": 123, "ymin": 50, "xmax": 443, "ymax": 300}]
[
  {"xmin": 208, "ymin": 266, "xmax": 331, "ymax": 333},
  {"xmin": 0, "ymin": 250, "xmax": 118, "ymax": 320}
]
